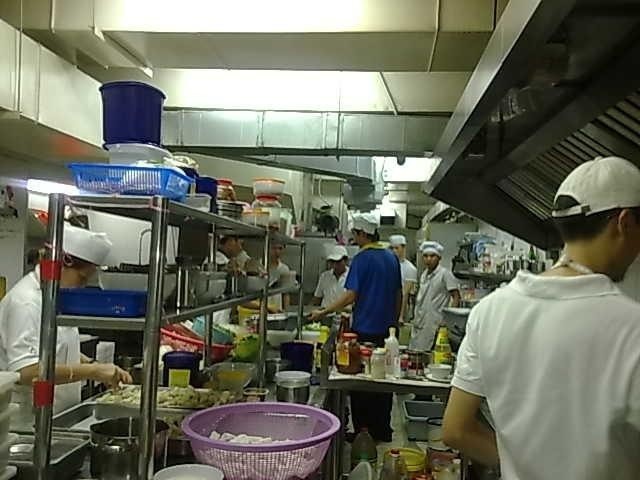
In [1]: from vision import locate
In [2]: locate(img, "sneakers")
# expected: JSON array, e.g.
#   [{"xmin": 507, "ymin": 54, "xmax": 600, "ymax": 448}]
[
  {"xmin": 380, "ymin": 433, "xmax": 391, "ymax": 442},
  {"xmin": 345, "ymin": 433, "xmax": 376, "ymax": 442}
]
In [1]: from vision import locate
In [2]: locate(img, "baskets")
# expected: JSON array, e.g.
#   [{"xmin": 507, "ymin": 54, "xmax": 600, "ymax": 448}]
[
  {"xmin": 181, "ymin": 402, "xmax": 341, "ymax": 480},
  {"xmin": 193, "ymin": 318, "xmax": 234, "ymax": 344},
  {"xmin": 160, "ymin": 328, "xmax": 235, "ymax": 362},
  {"xmin": 57, "ymin": 288, "xmax": 147, "ymax": 316},
  {"xmin": 66, "ymin": 162, "xmax": 196, "ymax": 201}
]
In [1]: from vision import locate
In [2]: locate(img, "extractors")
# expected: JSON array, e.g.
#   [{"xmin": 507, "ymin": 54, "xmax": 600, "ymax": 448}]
[{"xmin": 418, "ymin": 0, "xmax": 640, "ymax": 252}]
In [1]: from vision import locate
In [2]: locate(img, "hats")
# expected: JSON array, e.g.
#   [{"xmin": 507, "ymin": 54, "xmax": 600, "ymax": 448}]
[
  {"xmin": 325, "ymin": 246, "xmax": 348, "ymax": 261},
  {"xmin": 553, "ymin": 157, "xmax": 640, "ymax": 218},
  {"xmin": 63, "ymin": 221, "xmax": 112, "ymax": 266},
  {"xmin": 351, "ymin": 214, "xmax": 377, "ymax": 235},
  {"xmin": 420, "ymin": 241, "xmax": 444, "ymax": 258},
  {"xmin": 388, "ymin": 235, "xmax": 406, "ymax": 247}
]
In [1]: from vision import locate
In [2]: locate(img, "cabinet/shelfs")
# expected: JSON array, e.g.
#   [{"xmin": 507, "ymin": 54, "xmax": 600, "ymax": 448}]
[{"xmin": 33, "ymin": 192, "xmax": 307, "ymax": 479}]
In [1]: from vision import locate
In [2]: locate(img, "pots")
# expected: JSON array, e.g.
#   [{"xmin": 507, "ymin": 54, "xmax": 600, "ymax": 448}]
[
  {"xmin": 88, "ymin": 417, "xmax": 170, "ymax": 480},
  {"xmin": 276, "ymin": 382, "xmax": 309, "ymax": 404}
]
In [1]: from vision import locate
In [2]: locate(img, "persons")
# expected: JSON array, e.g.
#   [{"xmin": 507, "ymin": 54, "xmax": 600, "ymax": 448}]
[
  {"xmin": 198, "ymin": 232, "xmax": 245, "ymax": 325},
  {"xmin": 388, "ymin": 234, "xmax": 418, "ymax": 327},
  {"xmin": 1, "ymin": 221, "xmax": 134, "ymax": 420},
  {"xmin": 407, "ymin": 240, "xmax": 463, "ymax": 402},
  {"xmin": 440, "ymin": 154, "xmax": 640, "ymax": 480},
  {"xmin": 306, "ymin": 245, "xmax": 354, "ymax": 329},
  {"xmin": 266, "ymin": 235, "xmax": 291, "ymax": 312},
  {"xmin": 306, "ymin": 212, "xmax": 404, "ymax": 444}
]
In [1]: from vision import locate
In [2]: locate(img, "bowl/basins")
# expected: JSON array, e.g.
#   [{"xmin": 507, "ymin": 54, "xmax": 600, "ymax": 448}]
[
  {"xmin": 153, "ymin": 463, "xmax": 223, "ymax": 480},
  {"xmin": 206, "ymin": 363, "xmax": 256, "ymax": 390},
  {"xmin": 348, "ymin": 460, "xmax": 378, "ymax": 479}
]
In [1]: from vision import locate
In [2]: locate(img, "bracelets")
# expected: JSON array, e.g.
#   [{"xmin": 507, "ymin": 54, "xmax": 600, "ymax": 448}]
[{"xmin": 67, "ymin": 364, "xmax": 73, "ymax": 384}]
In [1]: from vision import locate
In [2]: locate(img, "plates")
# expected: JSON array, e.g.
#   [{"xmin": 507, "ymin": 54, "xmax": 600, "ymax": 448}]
[
  {"xmin": 217, "ymin": 199, "xmax": 246, "ymax": 222},
  {"xmin": 425, "ymin": 371, "xmax": 455, "ymax": 384}
]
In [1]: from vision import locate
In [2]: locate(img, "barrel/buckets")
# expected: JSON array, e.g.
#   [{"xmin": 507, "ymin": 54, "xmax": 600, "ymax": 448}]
[
  {"xmin": 380, "ymin": 450, "xmax": 408, "ymax": 479},
  {"xmin": 98, "ymin": 81, "xmax": 165, "ymax": 142}
]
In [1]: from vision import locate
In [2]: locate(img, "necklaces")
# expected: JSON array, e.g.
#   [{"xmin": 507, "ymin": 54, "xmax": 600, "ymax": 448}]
[{"xmin": 552, "ymin": 255, "xmax": 595, "ymax": 274}]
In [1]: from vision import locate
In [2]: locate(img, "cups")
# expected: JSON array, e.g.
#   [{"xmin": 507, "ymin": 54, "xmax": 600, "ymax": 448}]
[{"xmin": 430, "ymin": 363, "xmax": 454, "ymax": 379}]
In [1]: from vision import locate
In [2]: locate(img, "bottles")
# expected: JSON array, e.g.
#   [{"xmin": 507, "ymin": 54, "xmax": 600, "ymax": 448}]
[
  {"xmin": 379, "ymin": 447, "xmax": 412, "ymax": 479},
  {"xmin": 334, "ymin": 333, "xmax": 361, "ymax": 374},
  {"xmin": 402, "ymin": 435, "xmax": 423, "ymax": 480},
  {"xmin": 360, "ymin": 350, "xmax": 373, "ymax": 374},
  {"xmin": 382, "ymin": 327, "xmax": 401, "ymax": 378},
  {"xmin": 434, "ymin": 328, "xmax": 452, "ymax": 366},
  {"xmin": 372, "ymin": 352, "xmax": 388, "ymax": 378},
  {"xmin": 406, "ymin": 349, "xmax": 425, "ymax": 379}
]
